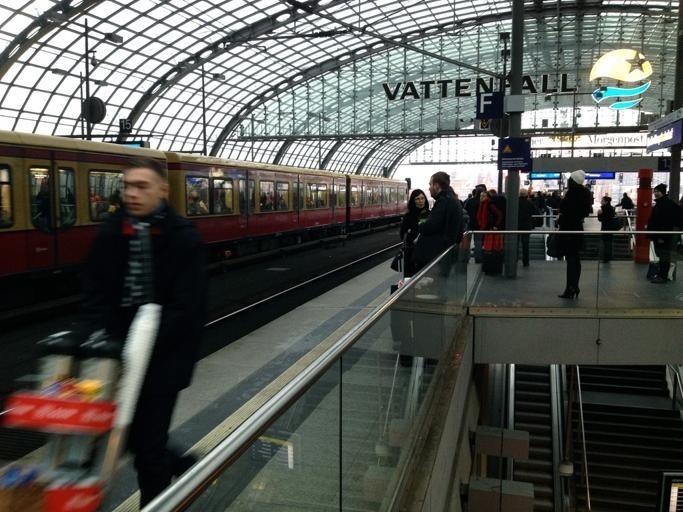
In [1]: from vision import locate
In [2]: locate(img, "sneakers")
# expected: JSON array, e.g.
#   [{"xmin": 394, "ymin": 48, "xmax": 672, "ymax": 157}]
[{"xmin": 651, "ymin": 278, "xmax": 667, "ymax": 284}]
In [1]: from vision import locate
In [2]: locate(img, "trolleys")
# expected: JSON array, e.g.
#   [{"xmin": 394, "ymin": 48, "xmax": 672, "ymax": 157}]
[{"xmin": 2, "ymin": 330, "xmax": 123, "ymax": 512}]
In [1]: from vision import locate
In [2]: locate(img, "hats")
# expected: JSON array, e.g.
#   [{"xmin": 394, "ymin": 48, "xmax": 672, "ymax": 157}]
[
  {"xmin": 654, "ymin": 183, "xmax": 667, "ymax": 194},
  {"xmin": 570, "ymin": 169, "xmax": 585, "ymax": 185}
]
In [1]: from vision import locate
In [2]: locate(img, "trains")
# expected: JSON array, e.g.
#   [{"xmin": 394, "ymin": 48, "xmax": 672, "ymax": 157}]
[{"xmin": 0, "ymin": 129, "xmax": 410, "ymax": 281}]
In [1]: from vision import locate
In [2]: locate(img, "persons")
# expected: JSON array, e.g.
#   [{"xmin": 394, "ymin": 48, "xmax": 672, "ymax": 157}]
[
  {"xmin": 90, "ymin": 160, "xmax": 197, "ymax": 512},
  {"xmin": 395, "ymin": 170, "xmax": 683, "ymax": 298}
]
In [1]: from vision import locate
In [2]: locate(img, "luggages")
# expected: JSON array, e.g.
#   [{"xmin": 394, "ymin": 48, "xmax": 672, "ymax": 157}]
[
  {"xmin": 482, "ymin": 249, "xmax": 503, "ymax": 273},
  {"xmin": 391, "ymin": 249, "xmax": 446, "ymax": 366}
]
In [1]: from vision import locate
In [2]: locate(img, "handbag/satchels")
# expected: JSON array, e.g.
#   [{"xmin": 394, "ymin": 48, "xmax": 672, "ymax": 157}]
[
  {"xmin": 546, "ymin": 233, "xmax": 564, "ymax": 258},
  {"xmin": 613, "ymin": 215, "xmax": 626, "ymax": 231},
  {"xmin": 647, "ymin": 261, "xmax": 677, "ymax": 281},
  {"xmin": 483, "ymin": 233, "xmax": 503, "ymax": 250}
]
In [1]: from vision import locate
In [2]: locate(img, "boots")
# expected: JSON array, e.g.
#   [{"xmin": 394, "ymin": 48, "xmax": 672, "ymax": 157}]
[{"xmin": 558, "ymin": 260, "xmax": 581, "ymax": 299}]
